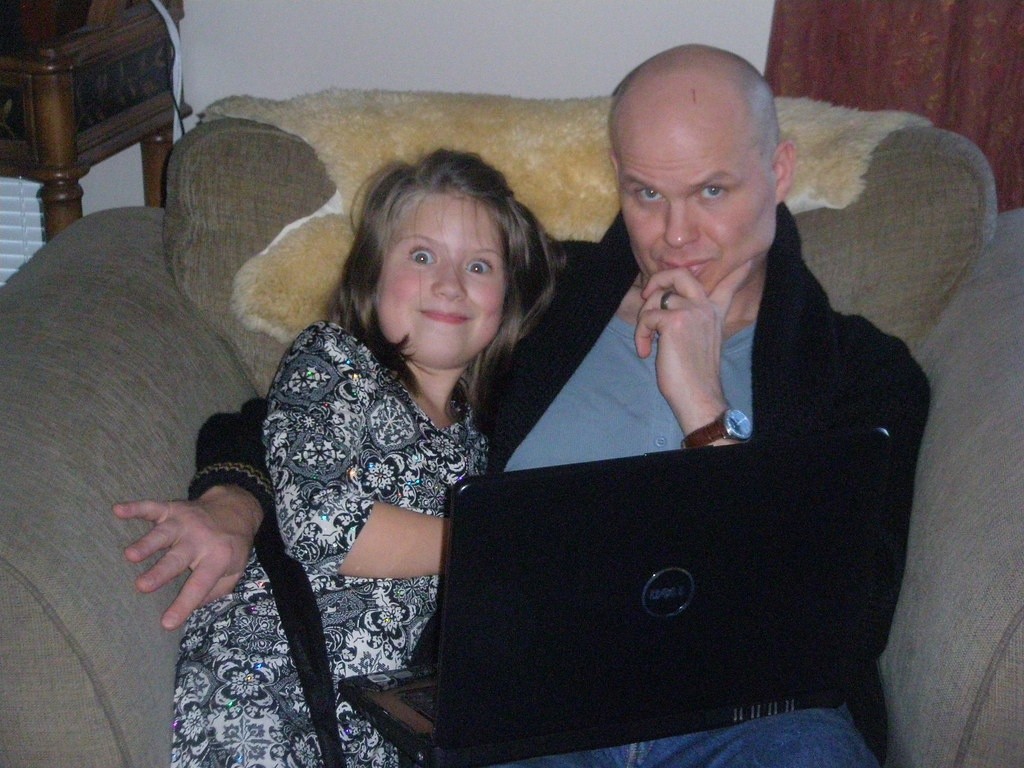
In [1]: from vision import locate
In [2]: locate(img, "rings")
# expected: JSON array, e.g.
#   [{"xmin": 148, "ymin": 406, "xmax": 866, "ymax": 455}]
[{"xmin": 659, "ymin": 291, "xmax": 674, "ymax": 309}]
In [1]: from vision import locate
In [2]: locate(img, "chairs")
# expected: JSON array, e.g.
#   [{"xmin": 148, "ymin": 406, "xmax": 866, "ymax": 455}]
[{"xmin": 0, "ymin": 0, "xmax": 192, "ymax": 247}]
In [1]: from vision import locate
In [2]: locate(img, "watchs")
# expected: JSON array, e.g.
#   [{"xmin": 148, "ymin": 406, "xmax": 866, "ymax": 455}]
[{"xmin": 680, "ymin": 408, "xmax": 753, "ymax": 449}]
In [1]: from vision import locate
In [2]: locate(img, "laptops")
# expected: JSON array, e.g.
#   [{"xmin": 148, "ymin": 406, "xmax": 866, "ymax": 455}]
[{"xmin": 337, "ymin": 425, "xmax": 889, "ymax": 767}]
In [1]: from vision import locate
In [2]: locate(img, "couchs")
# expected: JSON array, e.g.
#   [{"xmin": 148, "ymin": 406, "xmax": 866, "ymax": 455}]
[{"xmin": 0, "ymin": 91, "xmax": 1024, "ymax": 768}]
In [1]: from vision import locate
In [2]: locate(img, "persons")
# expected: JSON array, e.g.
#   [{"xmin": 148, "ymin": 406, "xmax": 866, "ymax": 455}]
[
  {"xmin": 169, "ymin": 146, "xmax": 567, "ymax": 768},
  {"xmin": 114, "ymin": 42, "xmax": 931, "ymax": 768}
]
